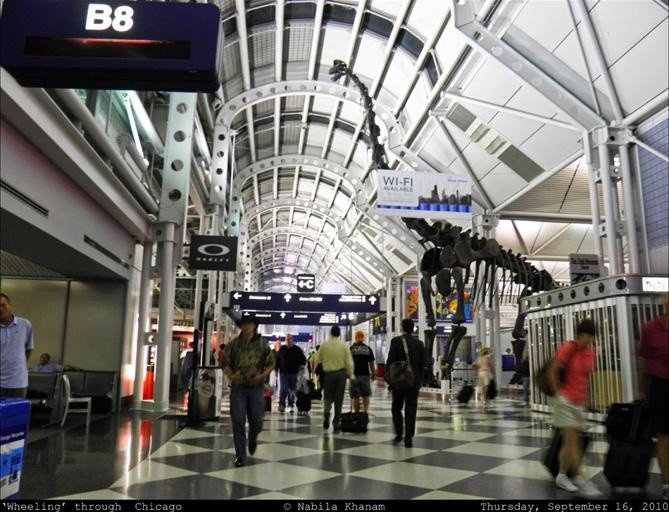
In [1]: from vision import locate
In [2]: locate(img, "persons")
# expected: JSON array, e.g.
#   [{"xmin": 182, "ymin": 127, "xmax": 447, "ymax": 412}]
[
  {"xmin": 1, "ymin": 293, "xmax": 34, "ymax": 399},
  {"xmin": 179, "ymin": 315, "xmax": 357, "ymax": 468},
  {"xmin": 634, "ymin": 293, "xmax": 668, "ymax": 491},
  {"xmin": 471, "ymin": 348, "xmax": 496, "ymax": 406},
  {"xmin": 30, "ymin": 352, "xmax": 82, "ymax": 374},
  {"xmin": 546, "ymin": 318, "xmax": 599, "ymax": 492},
  {"xmin": 386, "ymin": 319, "xmax": 426, "ymax": 448},
  {"xmin": 517, "ymin": 355, "xmax": 530, "ymax": 405},
  {"xmin": 350, "ymin": 331, "xmax": 378, "ymax": 413},
  {"xmin": 504, "ymin": 347, "xmax": 511, "ymax": 354}
]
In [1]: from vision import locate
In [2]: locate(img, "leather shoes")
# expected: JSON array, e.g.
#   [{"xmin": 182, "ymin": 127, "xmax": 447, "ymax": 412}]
[
  {"xmin": 234, "ymin": 458, "xmax": 244, "ymax": 466},
  {"xmin": 249, "ymin": 441, "xmax": 256, "ymax": 455}
]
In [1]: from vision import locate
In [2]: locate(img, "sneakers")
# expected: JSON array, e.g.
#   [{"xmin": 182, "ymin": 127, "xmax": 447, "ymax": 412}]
[
  {"xmin": 404, "ymin": 435, "xmax": 412, "ymax": 447},
  {"xmin": 393, "ymin": 435, "xmax": 401, "ymax": 442}
]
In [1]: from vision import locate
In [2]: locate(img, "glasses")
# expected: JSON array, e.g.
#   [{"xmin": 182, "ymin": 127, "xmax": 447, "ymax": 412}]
[{"xmin": 201, "ymin": 374, "xmax": 215, "ymax": 384}]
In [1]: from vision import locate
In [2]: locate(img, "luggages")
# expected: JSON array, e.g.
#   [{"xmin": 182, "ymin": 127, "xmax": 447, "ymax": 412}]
[
  {"xmin": 604, "ymin": 395, "xmax": 654, "ymax": 491},
  {"xmin": 304, "ymin": 380, "xmax": 322, "ymax": 400},
  {"xmin": 457, "ymin": 367, "xmax": 478, "ymax": 403},
  {"xmin": 544, "ymin": 428, "xmax": 590, "ymax": 479},
  {"xmin": 263, "ymin": 396, "xmax": 271, "ymax": 412},
  {"xmin": 339, "ymin": 379, "xmax": 368, "ymax": 433},
  {"xmin": 296, "ymin": 391, "xmax": 311, "ymax": 415}
]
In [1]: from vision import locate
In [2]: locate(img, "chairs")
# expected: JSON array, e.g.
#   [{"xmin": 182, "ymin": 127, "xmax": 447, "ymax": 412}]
[{"xmin": 25, "ymin": 367, "xmax": 119, "ymax": 425}]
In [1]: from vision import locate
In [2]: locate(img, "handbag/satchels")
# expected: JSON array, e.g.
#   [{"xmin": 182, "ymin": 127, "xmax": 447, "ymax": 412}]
[
  {"xmin": 384, "ymin": 361, "xmax": 414, "ymax": 388},
  {"xmin": 536, "ymin": 358, "xmax": 567, "ymax": 395},
  {"xmin": 487, "ymin": 380, "xmax": 497, "ymax": 399},
  {"xmin": 606, "ymin": 403, "xmax": 656, "ymax": 439}
]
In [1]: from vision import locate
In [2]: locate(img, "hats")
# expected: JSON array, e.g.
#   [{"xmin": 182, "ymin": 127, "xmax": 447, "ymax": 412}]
[
  {"xmin": 355, "ymin": 331, "xmax": 364, "ymax": 342},
  {"xmin": 479, "ymin": 347, "xmax": 489, "ymax": 356},
  {"xmin": 235, "ymin": 315, "xmax": 260, "ymax": 328}
]
